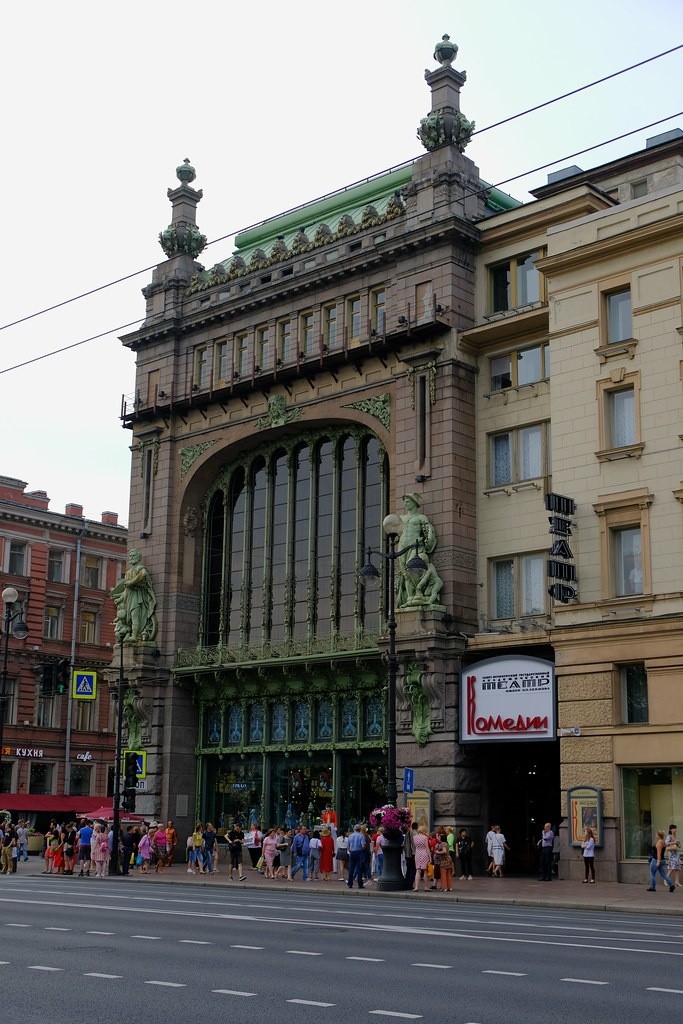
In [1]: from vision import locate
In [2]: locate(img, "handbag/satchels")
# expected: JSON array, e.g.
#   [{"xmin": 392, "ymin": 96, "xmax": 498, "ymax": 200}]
[{"xmin": 666, "ymin": 836, "xmax": 678, "ymax": 851}]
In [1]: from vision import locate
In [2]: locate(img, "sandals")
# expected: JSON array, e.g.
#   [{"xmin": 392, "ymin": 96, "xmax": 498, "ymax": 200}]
[
  {"xmin": 499, "ymin": 873, "xmax": 505, "ymax": 877},
  {"xmin": 486, "ymin": 870, "xmax": 491, "ymax": 878},
  {"xmin": 493, "ymin": 870, "xmax": 499, "ymax": 878}
]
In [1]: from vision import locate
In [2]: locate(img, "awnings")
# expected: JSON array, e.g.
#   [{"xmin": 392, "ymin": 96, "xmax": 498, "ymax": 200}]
[{"xmin": 0, "ymin": 793, "xmax": 113, "ymax": 813}]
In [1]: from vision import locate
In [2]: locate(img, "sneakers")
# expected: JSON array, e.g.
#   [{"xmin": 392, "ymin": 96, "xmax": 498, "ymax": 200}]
[
  {"xmin": 468, "ymin": 875, "xmax": 473, "ymax": 881},
  {"xmin": 669, "ymin": 885, "xmax": 675, "ymax": 892},
  {"xmin": 363, "ymin": 880, "xmax": 373, "ymax": 886},
  {"xmin": 646, "ymin": 887, "xmax": 656, "ymax": 892},
  {"xmin": 239, "ymin": 875, "xmax": 247, "ymax": 882},
  {"xmin": 228, "ymin": 875, "xmax": 235, "ymax": 881},
  {"xmin": 459, "ymin": 875, "xmax": 465, "ymax": 881}
]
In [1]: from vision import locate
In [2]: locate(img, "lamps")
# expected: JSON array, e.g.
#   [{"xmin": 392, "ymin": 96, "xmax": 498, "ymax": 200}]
[
  {"xmin": 276, "ymin": 358, "xmax": 283, "ymax": 366},
  {"xmin": 233, "ymin": 371, "xmax": 240, "ymax": 378},
  {"xmin": 254, "ymin": 365, "xmax": 262, "ymax": 372},
  {"xmin": 397, "ymin": 315, "xmax": 408, "ymax": 323},
  {"xmin": 158, "ymin": 390, "xmax": 166, "ymax": 397},
  {"xmin": 322, "ymin": 344, "xmax": 329, "ymax": 351},
  {"xmin": 298, "ymin": 351, "xmax": 305, "ymax": 358},
  {"xmin": 192, "ymin": 383, "xmax": 199, "ymax": 391},
  {"xmin": 135, "ymin": 397, "xmax": 144, "ymax": 404},
  {"xmin": 371, "ymin": 328, "xmax": 378, "ymax": 336},
  {"xmin": 435, "ymin": 304, "xmax": 444, "ymax": 312}
]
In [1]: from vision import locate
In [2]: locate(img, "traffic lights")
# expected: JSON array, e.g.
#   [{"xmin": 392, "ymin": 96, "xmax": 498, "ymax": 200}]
[
  {"xmin": 127, "ymin": 789, "xmax": 137, "ymax": 812},
  {"xmin": 124, "ymin": 752, "xmax": 140, "ymax": 787},
  {"xmin": 53, "ymin": 659, "xmax": 69, "ymax": 691}
]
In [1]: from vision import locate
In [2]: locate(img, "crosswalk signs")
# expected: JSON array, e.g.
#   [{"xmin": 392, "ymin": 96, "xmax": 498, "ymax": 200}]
[{"xmin": 74, "ymin": 672, "xmax": 96, "ymax": 699}]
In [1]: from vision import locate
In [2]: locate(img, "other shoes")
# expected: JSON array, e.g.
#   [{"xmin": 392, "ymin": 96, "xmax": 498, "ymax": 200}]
[
  {"xmin": 583, "ymin": 880, "xmax": 588, "ymax": 884},
  {"xmin": 140, "ymin": 863, "xmax": 172, "ymax": 874},
  {"xmin": 590, "ymin": 879, "xmax": 596, "ymax": 884},
  {"xmin": 0, "ymin": 869, "xmax": 6, "ymax": 874},
  {"xmin": 43, "ymin": 867, "xmax": 108, "ymax": 877},
  {"xmin": 188, "ymin": 867, "xmax": 220, "ymax": 875},
  {"xmin": 537, "ymin": 877, "xmax": 545, "ymax": 881},
  {"xmin": 413, "ymin": 885, "xmax": 453, "ymax": 893},
  {"xmin": 542, "ymin": 877, "xmax": 552, "ymax": 881},
  {"xmin": 263, "ymin": 871, "xmax": 345, "ymax": 881},
  {"xmin": 4, "ymin": 871, "xmax": 11, "ymax": 875}
]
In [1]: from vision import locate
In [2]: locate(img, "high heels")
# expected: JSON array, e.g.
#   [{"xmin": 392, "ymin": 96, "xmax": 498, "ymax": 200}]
[
  {"xmin": 663, "ymin": 880, "xmax": 668, "ymax": 887},
  {"xmin": 675, "ymin": 881, "xmax": 683, "ymax": 888}
]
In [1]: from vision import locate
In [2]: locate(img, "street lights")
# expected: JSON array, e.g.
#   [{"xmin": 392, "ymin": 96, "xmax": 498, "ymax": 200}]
[
  {"xmin": 357, "ymin": 514, "xmax": 429, "ymax": 893},
  {"xmin": 0, "ymin": 587, "xmax": 30, "ymax": 757}
]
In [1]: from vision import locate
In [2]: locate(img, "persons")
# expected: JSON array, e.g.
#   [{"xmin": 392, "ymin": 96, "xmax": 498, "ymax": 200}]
[
  {"xmin": 0, "ymin": 817, "xmax": 29, "ymax": 875},
  {"xmin": 401, "ymin": 822, "xmax": 473, "ymax": 892},
  {"xmin": 41, "ymin": 819, "xmax": 178, "ymax": 877},
  {"xmin": 580, "ymin": 827, "xmax": 596, "ymax": 883},
  {"xmin": 485, "ymin": 825, "xmax": 510, "ymax": 879},
  {"xmin": 187, "ymin": 822, "xmax": 219, "ymax": 875},
  {"xmin": 224, "ymin": 822, "xmax": 388, "ymax": 889},
  {"xmin": 323, "ymin": 804, "xmax": 337, "ymax": 830},
  {"xmin": 113, "ymin": 550, "xmax": 156, "ymax": 642},
  {"xmin": 663, "ymin": 825, "xmax": 683, "ymax": 887},
  {"xmin": 646, "ymin": 832, "xmax": 675, "ymax": 892},
  {"xmin": 397, "ymin": 493, "xmax": 444, "ymax": 605},
  {"xmin": 537, "ymin": 823, "xmax": 554, "ymax": 881},
  {"xmin": 266, "ymin": 394, "xmax": 288, "ymax": 428}
]
